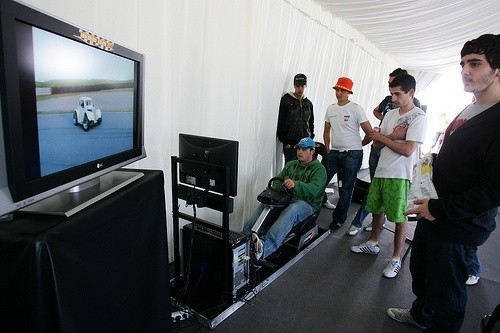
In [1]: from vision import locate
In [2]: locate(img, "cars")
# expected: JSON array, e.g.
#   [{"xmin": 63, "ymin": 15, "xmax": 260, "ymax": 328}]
[{"xmin": 72, "ymin": 98, "xmax": 102, "ymax": 130}]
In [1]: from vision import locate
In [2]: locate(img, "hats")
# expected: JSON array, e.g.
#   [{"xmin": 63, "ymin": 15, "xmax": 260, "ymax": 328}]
[
  {"xmin": 332, "ymin": 77, "xmax": 353, "ymax": 94},
  {"xmin": 294, "ymin": 74, "xmax": 307, "ymax": 85},
  {"xmin": 294, "ymin": 138, "xmax": 315, "ymax": 150}
]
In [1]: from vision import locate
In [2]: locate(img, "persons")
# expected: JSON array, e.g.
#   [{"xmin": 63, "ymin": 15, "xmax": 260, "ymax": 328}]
[
  {"xmin": 351, "ymin": 74, "xmax": 427, "ymax": 278},
  {"xmin": 387, "ymin": 33, "xmax": 500, "ymax": 333},
  {"xmin": 277, "ymin": 74, "xmax": 336, "ymax": 209},
  {"xmin": 237, "ymin": 137, "xmax": 327, "ymax": 262},
  {"xmin": 321, "ymin": 78, "xmax": 373, "ymax": 230},
  {"xmin": 347, "ymin": 68, "xmax": 420, "ymax": 236}
]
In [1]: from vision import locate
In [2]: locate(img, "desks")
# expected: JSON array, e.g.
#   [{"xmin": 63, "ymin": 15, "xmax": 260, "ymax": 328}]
[{"xmin": 0, "ymin": 167, "xmax": 174, "ymax": 333}]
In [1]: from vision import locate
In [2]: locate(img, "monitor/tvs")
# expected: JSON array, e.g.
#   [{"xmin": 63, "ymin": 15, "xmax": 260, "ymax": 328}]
[
  {"xmin": 1, "ymin": 0, "xmax": 147, "ymax": 219},
  {"xmin": 179, "ymin": 133, "xmax": 239, "ymax": 197}
]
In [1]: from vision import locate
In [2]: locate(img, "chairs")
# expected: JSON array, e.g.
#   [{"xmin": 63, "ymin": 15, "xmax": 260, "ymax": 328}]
[
  {"xmin": 262, "ymin": 142, "xmax": 328, "ymax": 238},
  {"xmin": 382, "ymin": 213, "xmax": 418, "ymax": 263}
]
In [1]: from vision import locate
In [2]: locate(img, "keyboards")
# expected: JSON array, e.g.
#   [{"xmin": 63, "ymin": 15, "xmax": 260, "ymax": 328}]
[{"xmin": 183, "ymin": 222, "xmax": 246, "ymax": 246}]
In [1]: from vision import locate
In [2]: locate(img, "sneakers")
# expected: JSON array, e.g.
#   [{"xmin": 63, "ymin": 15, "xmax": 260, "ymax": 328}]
[
  {"xmin": 365, "ymin": 221, "xmax": 386, "ymax": 231},
  {"xmin": 386, "ymin": 308, "xmax": 426, "ymax": 328},
  {"xmin": 351, "ymin": 241, "xmax": 380, "ymax": 254},
  {"xmin": 322, "ymin": 200, "xmax": 337, "ymax": 209},
  {"xmin": 382, "ymin": 258, "xmax": 402, "ymax": 278},
  {"xmin": 346, "ymin": 225, "xmax": 361, "ymax": 236},
  {"xmin": 466, "ymin": 274, "xmax": 479, "ymax": 285},
  {"xmin": 328, "ymin": 221, "xmax": 343, "ymax": 230},
  {"xmin": 252, "ymin": 233, "xmax": 263, "ymax": 260}
]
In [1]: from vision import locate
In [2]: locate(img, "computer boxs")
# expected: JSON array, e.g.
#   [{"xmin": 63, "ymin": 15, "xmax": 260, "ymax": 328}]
[{"xmin": 181, "ymin": 228, "xmax": 250, "ymax": 295}]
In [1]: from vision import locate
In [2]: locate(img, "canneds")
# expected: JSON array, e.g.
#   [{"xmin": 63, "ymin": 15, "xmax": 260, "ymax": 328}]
[{"xmin": 373, "ymin": 126, "xmax": 382, "ymax": 133}]
